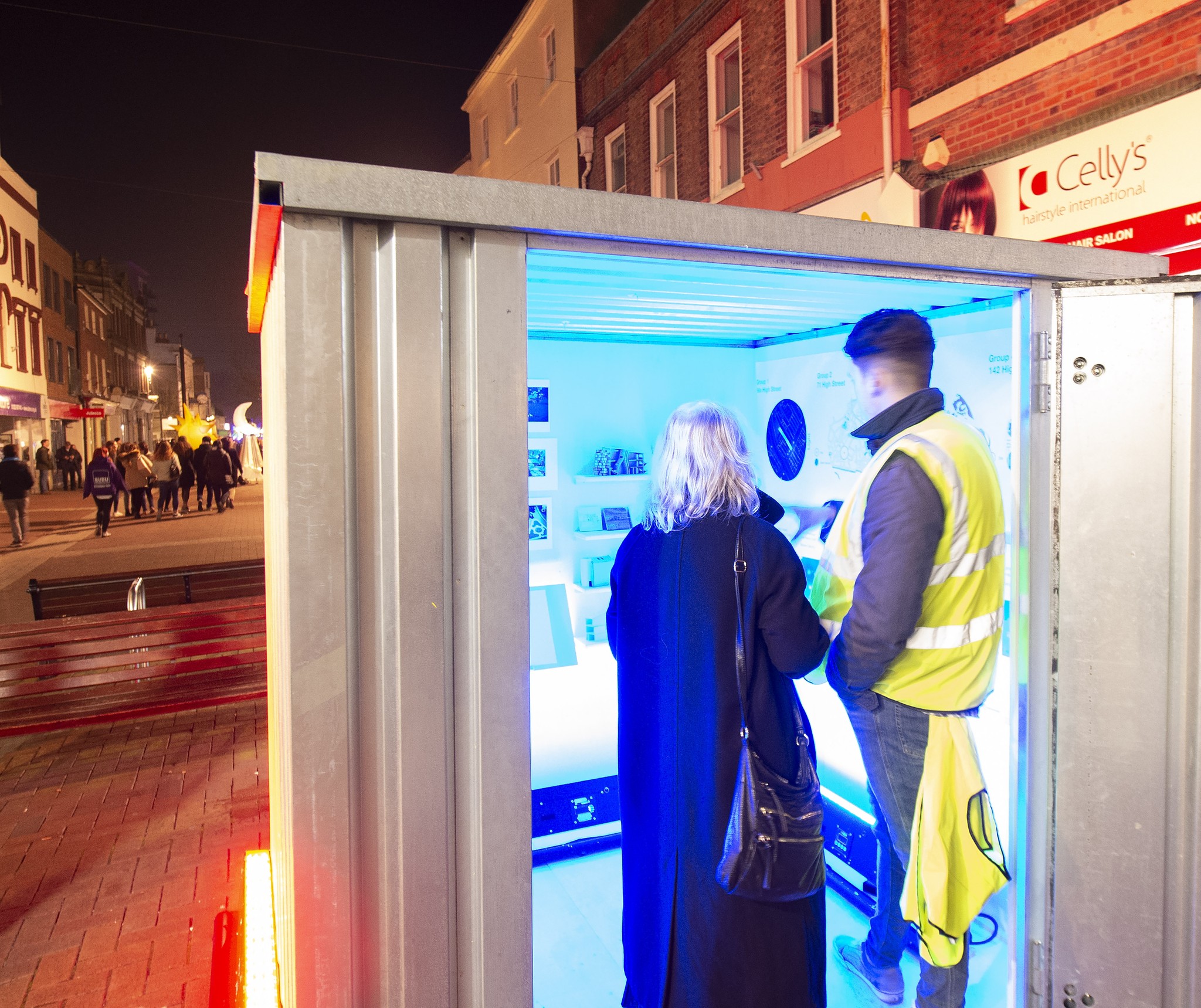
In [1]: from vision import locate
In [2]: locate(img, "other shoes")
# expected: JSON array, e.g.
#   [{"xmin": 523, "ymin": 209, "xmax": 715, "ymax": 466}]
[
  {"xmin": 112, "ymin": 491, "xmax": 235, "ymax": 521},
  {"xmin": 102, "ymin": 532, "xmax": 111, "ymax": 537},
  {"xmin": 79, "ymin": 484, "xmax": 84, "ymax": 488},
  {"xmin": 96, "ymin": 525, "xmax": 101, "ymax": 536},
  {"xmin": 71, "ymin": 487, "xmax": 78, "ymax": 491},
  {"xmin": 10, "ymin": 540, "xmax": 22, "ymax": 547},
  {"xmin": 41, "ymin": 491, "xmax": 49, "ymax": 495}
]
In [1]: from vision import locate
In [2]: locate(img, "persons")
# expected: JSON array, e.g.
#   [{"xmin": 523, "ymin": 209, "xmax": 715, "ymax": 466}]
[
  {"xmin": 36, "ymin": 439, "xmax": 53, "ymax": 494},
  {"xmin": 607, "ymin": 400, "xmax": 830, "ymax": 1008},
  {"xmin": 83, "ymin": 447, "xmax": 127, "ymax": 537},
  {"xmin": 934, "ymin": 170, "xmax": 996, "ymax": 236},
  {"xmin": 54, "ymin": 436, "xmax": 243, "ymax": 522},
  {"xmin": 0, "ymin": 444, "xmax": 34, "ymax": 547},
  {"xmin": 805, "ymin": 308, "xmax": 1005, "ymax": 1008}
]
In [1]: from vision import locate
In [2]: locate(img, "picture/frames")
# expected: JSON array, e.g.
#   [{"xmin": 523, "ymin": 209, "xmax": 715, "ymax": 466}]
[
  {"xmin": 528, "ymin": 437, "xmax": 558, "ymax": 490},
  {"xmin": 528, "ymin": 497, "xmax": 553, "ymax": 551}
]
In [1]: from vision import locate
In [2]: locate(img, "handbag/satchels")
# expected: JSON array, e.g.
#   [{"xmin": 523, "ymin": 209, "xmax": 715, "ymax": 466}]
[
  {"xmin": 170, "ymin": 454, "xmax": 179, "ymax": 477},
  {"xmin": 136, "ymin": 457, "xmax": 152, "ymax": 477},
  {"xmin": 712, "ymin": 733, "xmax": 823, "ymax": 902},
  {"xmin": 224, "ymin": 474, "xmax": 234, "ymax": 485}
]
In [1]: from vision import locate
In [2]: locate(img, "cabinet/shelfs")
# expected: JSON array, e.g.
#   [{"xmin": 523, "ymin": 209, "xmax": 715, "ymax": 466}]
[{"xmin": 572, "ymin": 474, "xmax": 651, "ymax": 645}]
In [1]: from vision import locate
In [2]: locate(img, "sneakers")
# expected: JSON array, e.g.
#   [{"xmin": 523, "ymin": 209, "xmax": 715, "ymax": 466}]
[{"xmin": 832, "ymin": 936, "xmax": 905, "ymax": 1003}]
